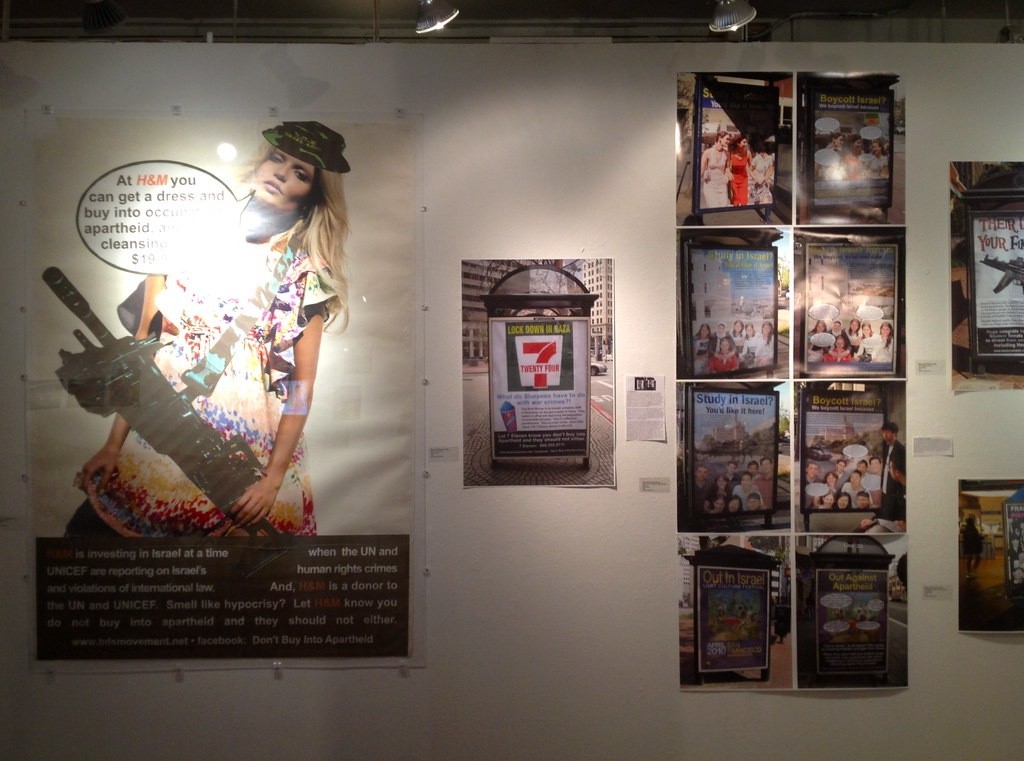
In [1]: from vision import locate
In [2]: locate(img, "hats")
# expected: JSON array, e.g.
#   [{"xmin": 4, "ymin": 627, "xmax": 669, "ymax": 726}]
[{"xmin": 262, "ymin": 121, "xmax": 350, "ymax": 173}]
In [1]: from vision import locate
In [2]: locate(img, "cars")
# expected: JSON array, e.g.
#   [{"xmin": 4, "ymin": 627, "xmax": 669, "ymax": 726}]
[{"xmin": 591, "ymin": 363, "xmax": 607, "ymax": 376}]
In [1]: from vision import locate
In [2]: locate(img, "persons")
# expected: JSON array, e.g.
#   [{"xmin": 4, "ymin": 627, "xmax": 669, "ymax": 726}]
[
  {"xmin": 879, "ymin": 422, "xmax": 904, "ymax": 495},
  {"xmin": 860, "ymin": 449, "xmax": 906, "ymax": 531},
  {"xmin": 694, "ymin": 457, "xmax": 774, "ymax": 514},
  {"xmin": 699, "ymin": 131, "xmax": 775, "ymax": 209},
  {"xmin": 804, "ymin": 457, "xmax": 882, "ymax": 509},
  {"xmin": 814, "ymin": 131, "xmax": 889, "ymax": 181},
  {"xmin": 63, "ymin": 120, "xmax": 352, "ymax": 537},
  {"xmin": 693, "ymin": 320, "xmax": 775, "ymax": 375},
  {"xmin": 807, "ymin": 318, "xmax": 893, "ymax": 363},
  {"xmin": 963, "ymin": 518, "xmax": 981, "ymax": 580}
]
